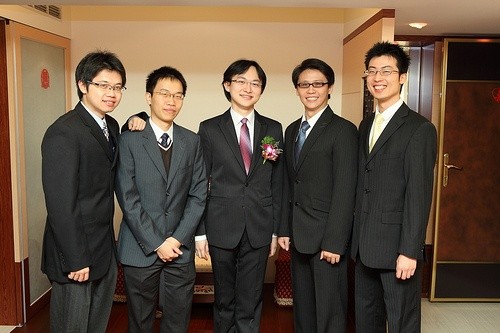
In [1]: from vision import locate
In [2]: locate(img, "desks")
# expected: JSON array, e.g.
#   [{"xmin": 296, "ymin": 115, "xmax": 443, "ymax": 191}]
[{"xmin": 194, "ymin": 240, "xmax": 215, "ymax": 303}]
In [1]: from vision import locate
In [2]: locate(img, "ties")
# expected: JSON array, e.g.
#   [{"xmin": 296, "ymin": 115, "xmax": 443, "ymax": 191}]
[
  {"xmin": 238, "ymin": 118, "xmax": 252, "ymax": 175},
  {"xmin": 102, "ymin": 121, "xmax": 110, "ymax": 143},
  {"xmin": 370, "ymin": 113, "xmax": 383, "ymax": 154},
  {"xmin": 160, "ymin": 133, "xmax": 170, "ymax": 149},
  {"xmin": 292, "ymin": 120, "xmax": 310, "ymax": 166}
]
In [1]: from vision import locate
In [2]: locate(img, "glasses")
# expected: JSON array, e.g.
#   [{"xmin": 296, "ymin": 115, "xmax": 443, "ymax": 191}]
[
  {"xmin": 151, "ymin": 91, "xmax": 185, "ymax": 100},
  {"xmin": 363, "ymin": 67, "xmax": 399, "ymax": 76},
  {"xmin": 296, "ymin": 81, "xmax": 328, "ymax": 88},
  {"xmin": 86, "ymin": 80, "xmax": 127, "ymax": 95},
  {"xmin": 230, "ymin": 78, "xmax": 263, "ymax": 88}
]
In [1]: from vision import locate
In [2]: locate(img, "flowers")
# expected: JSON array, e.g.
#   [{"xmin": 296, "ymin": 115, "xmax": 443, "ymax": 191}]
[{"xmin": 260, "ymin": 136, "xmax": 283, "ymax": 164}]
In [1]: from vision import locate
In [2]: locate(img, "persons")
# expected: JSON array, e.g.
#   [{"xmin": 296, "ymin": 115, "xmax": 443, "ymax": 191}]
[
  {"xmin": 285, "ymin": 59, "xmax": 359, "ymax": 333},
  {"xmin": 200, "ymin": 60, "xmax": 284, "ymax": 333},
  {"xmin": 41, "ymin": 52, "xmax": 149, "ymax": 333},
  {"xmin": 116, "ymin": 67, "xmax": 208, "ymax": 333},
  {"xmin": 351, "ymin": 41, "xmax": 437, "ymax": 333}
]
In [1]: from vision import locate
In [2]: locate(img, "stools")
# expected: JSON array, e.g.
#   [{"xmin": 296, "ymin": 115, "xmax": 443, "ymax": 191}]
[{"xmin": 274, "ymin": 245, "xmax": 292, "ymax": 308}]
